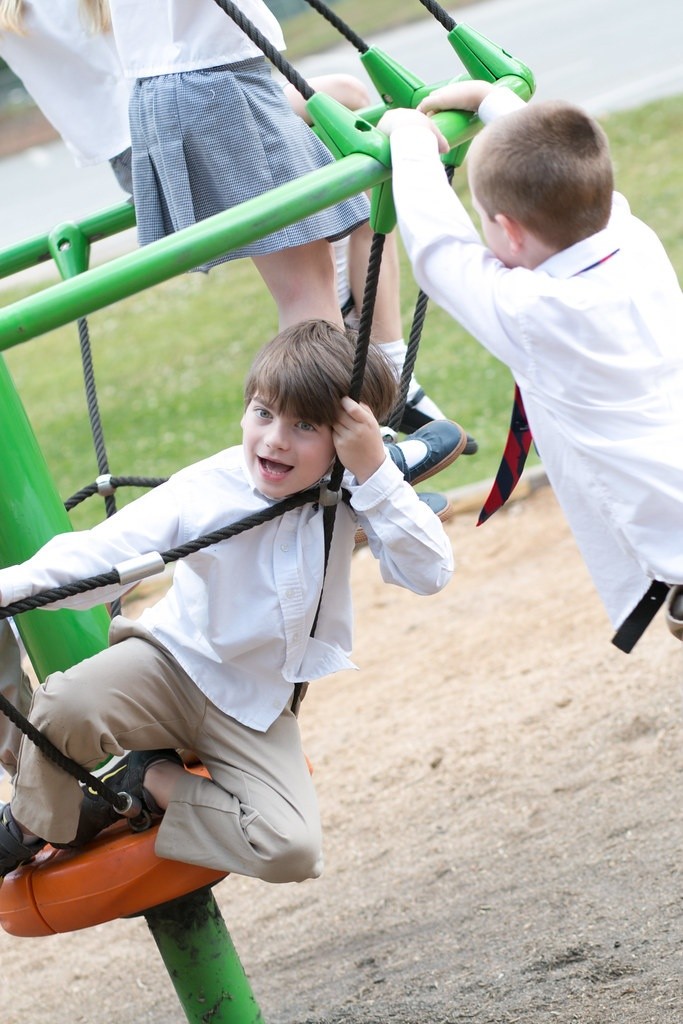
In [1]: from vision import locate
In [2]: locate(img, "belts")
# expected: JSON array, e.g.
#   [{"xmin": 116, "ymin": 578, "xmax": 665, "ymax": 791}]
[{"xmin": 612, "ymin": 580, "xmax": 672, "ymax": 655}]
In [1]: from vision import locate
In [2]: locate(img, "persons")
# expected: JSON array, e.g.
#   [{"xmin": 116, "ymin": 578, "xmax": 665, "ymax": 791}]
[
  {"xmin": 109, "ymin": 0, "xmax": 467, "ymax": 523},
  {"xmin": 0, "ymin": 320, "xmax": 454, "ymax": 886},
  {"xmin": 1, "ymin": 1, "xmax": 479, "ymax": 457},
  {"xmin": 378, "ymin": 81, "xmax": 683, "ymax": 644}
]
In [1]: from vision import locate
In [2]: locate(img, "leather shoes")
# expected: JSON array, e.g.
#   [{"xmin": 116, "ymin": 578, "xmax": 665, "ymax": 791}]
[
  {"xmin": 356, "ymin": 418, "xmax": 468, "ymax": 506},
  {"xmin": 384, "ymin": 385, "xmax": 479, "ymax": 456}
]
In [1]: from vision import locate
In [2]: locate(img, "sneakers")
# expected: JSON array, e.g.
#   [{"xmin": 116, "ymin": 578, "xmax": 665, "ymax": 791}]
[
  {"xmin": 49, "ymin": 747, "xmax": 188, "ymax": 850},
  {"xmin": 0, "ymin": 802, "xmax": 47, "ymax": 881}
]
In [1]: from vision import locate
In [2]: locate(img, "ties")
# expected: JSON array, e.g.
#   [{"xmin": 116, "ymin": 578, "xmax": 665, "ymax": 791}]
[{"xmin": 476, "ymin": 379, "xmax": 533, "ymax": 527}]
